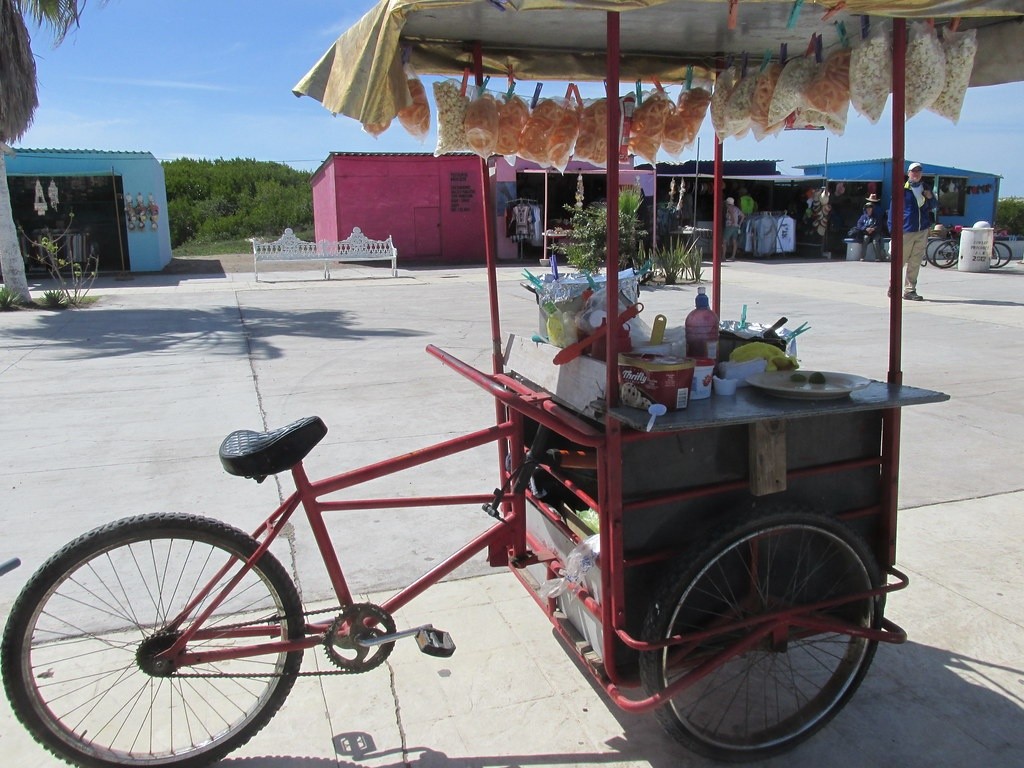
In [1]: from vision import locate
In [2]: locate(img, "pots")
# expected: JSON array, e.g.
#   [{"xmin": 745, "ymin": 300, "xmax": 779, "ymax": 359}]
[{"xmin": 521, "ymin": 259, "xmax": 652, "ymax": 344}]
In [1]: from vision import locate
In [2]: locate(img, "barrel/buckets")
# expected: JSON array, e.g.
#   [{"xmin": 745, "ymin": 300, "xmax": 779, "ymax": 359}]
[{"xmin": 958, "ymin": 228, "xmax": 994, "ymax": 273}]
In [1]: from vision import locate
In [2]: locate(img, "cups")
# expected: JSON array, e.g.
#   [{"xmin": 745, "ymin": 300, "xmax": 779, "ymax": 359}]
[{"xmin": 632, "ymin": 341, "xmax": 672, "ymax": 355}]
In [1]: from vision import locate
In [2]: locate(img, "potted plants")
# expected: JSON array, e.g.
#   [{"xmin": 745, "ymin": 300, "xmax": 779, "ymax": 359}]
[{"xmin": 653, "ymin": 233, "xmax": 700, "ymax": 285}]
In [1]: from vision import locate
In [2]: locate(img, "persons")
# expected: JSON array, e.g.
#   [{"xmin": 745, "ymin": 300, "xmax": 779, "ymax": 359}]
[
  {"xmin": 736, "ymin": 187, "xmax": 759, "ymax": 216},
  {"xmin": 848, "ymin": 193, "xmax": 884, "ymax": 262},
  {"xmin": 721, "ymin": 197, "xmax": 745, "ymax": 261},
  {"xmin": 887, "ymin": 162, "xmax": 938, "ymax": 301}
]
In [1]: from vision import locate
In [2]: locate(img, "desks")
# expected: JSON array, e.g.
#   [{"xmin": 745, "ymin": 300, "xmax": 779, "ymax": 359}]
[
  {"xmin": 510, "ymin": 361, "xmax": 952, "ymax": 435},
  {"xmin": 669, "ymin": 229, "xmax": 712, "ymax": 261},
  {"xmin": 542, "ymin": 233, "xmax": 570, "ymax": 265}
]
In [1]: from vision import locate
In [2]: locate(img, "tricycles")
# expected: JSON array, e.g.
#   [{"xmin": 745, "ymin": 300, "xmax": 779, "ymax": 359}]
[{"xmin": 2, "ymin": 0, "xmax": 1022, "ymax": 767}]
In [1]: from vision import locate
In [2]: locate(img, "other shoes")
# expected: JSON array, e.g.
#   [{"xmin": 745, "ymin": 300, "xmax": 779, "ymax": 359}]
[
  {"xmin": 860, "ymin": 256, "xmax": 865, "ymax": 261},
  {"xmin": 721, "ymin": 257, "xmax": 726, "ymax": 262},
  {"xmin": 875, "ymin": 259, "xmax": 880, "ymax": 262},
  {"xmin": 726, "ymin": 257, "xmax": 736, "ymax": 262}
]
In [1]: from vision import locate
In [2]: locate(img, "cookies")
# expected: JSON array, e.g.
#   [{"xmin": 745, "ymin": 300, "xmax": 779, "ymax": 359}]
[{"xmin": 621, "ymin": 382, "xmax": 652, "ymax": 410}]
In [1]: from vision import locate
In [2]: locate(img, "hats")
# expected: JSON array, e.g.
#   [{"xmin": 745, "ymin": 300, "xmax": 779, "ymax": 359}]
[
  {"xmin": 725, "ymin": 198, "xmax": 735, "ymax": 205},
  {"xmin": 931, "ymin": 224, "xmax": 945, "ymax": 237},
  {"xmin": 908, "ymin": 162, "xmax": 923, "ymax": 170},
  {"xmin": 865, "ymin": 193, "xmax": 880, "ymax": 202}
]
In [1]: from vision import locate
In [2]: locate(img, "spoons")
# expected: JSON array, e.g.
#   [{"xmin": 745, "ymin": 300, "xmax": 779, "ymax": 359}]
[{"xmin": 645, "ymin": 404, "xmax": 667, "ymax": 432}]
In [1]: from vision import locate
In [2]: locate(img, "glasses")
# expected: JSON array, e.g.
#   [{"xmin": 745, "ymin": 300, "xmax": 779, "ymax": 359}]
[{"xmin": 865, "ymin": 205, "xmax": 874, "ymax": 209}]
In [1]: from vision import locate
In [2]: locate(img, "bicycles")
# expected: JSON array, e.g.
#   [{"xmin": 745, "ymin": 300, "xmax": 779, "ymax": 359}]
[{"xmin": 924, "ymin": 224, "xmax": 1012, "ymax": 269}]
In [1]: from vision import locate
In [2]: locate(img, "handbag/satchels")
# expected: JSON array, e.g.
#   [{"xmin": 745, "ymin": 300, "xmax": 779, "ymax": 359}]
[{"xmin": 929, "ymin": 211, "xmax": 935, "ymax": 224}]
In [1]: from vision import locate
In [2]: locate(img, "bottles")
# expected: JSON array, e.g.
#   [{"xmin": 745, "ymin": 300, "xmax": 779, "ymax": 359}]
[
  {"xmin": 686, "ymin": 287, "xmax": 720, "ymax": 376},
  {"xmin": 591, "ymin": 317, "xmax": 606, "ymax": 362},
  {"xmin": 619, "ymin": 323, "xmax": 632, "ymax": 356}
]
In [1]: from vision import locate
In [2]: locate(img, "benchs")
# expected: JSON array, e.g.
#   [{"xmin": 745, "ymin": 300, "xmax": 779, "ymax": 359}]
[
  {"xmin": 994, "ymin": 235, "xmax": 1024, "ymax": 260},
  {"xmin": 324, "ymin": 226, "xmax": 398, "ymax": 280},
  {"xmin": 253, "ymin": 228, "xmax": 330, "ymax": 282},
  {"xmin": 844, "ymin": 236, "xmax": 947, "ymax": 261}
]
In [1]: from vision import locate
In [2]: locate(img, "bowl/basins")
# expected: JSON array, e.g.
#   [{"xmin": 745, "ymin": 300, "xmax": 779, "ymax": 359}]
[
  {"xmin": 714, "ymin": 380, "xmax": 736, "ymax": 395},
  {"xmin": 719, "ymin": 335, "xmax": 782, "ymax": 363},
  {"xmin": 616, "ymin": 353, "xmax": 696, "ymax": 411},
  {"xmin": 688, "ymin": 356, "xmax": 715, "ymax": 400}
]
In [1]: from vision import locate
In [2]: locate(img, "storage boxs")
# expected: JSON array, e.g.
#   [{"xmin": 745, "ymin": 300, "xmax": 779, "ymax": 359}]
[{"xmin": 617, "ymin": 352, "xmax": 694, "ymax": 412}]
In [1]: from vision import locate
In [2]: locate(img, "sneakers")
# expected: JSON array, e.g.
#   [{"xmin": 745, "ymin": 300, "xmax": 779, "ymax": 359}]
[
  {"xmin": 904, "ymin": 291, "xmax": 923, "ymax": 300},
  {"xmin": 888, "ymin": 287, "xmax": 891, "ymax": 297}
]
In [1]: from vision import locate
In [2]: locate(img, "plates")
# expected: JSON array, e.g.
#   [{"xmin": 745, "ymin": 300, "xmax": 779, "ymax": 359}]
[{"xmin": 746, "ymin": 372, "xmax": 870, "ymax": 400}]
